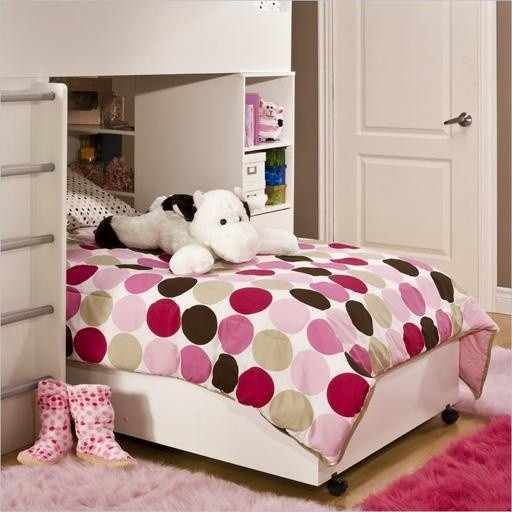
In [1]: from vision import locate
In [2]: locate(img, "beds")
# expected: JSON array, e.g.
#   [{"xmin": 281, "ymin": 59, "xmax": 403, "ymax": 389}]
[{"xmin": 66, "ymin": 216, "xmax": 500, "ymax": 496}]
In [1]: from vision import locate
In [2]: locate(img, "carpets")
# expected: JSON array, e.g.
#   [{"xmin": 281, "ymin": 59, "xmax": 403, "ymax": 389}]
[
  {"xmin": 0, "ymin": 454, "xmax": 363, "ymax": 512},
  {"xmin": 452, "ymin": 344, "xmax": 512, "ymax": 416},
  {"xmin": 352, "ymin": 415, "xmax": 512, "ymax": 512}
]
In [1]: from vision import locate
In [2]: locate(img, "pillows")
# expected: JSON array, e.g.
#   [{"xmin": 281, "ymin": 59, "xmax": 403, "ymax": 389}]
[{"xmin": 66, "ymin": 167, "xmax": 143, "ymax": 231}]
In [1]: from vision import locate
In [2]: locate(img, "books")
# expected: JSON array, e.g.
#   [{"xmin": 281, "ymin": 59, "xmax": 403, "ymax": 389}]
[{"xmin": 244, "ymin": 91, "xmax": 260, "ymax": 148}]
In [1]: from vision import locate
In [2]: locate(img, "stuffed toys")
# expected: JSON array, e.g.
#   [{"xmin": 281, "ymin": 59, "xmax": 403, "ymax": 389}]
[
  {"xmin": 93, "ymin": 184, "xmax": 301, "ymax": 280},
  {"xmin": 256, "ymin": 99, "xmax": 286, "ymax": 142}
]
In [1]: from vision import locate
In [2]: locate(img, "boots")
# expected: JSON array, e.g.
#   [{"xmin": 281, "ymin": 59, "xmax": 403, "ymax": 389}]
[
  {"xmin": 67, "ymin": 383, "xmax": 138, "ymax": 469},
  {"xmin": 16, "ymin": 378, "xmax": 73, "ymax": 465}
]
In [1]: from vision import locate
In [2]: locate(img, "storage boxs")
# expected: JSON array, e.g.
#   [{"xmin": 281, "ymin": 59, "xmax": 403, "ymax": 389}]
[{"xmin": 242, "ymin": 147, "xmax": 287, "ymax": 212}]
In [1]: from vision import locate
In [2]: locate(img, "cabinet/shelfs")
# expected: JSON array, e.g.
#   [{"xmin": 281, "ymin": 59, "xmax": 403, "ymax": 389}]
[
  {"xmin": 66, "ymin": 124, "xmax": 133, "ymax": 197},
  {"xmin": 134, "ymin": 74, "xmax": 296, "ymax": 214}
]
